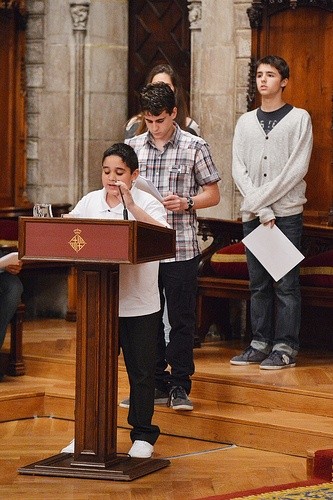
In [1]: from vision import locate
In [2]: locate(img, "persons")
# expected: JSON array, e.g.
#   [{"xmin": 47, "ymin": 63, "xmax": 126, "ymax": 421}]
[
  {"xmin": 59, "ymin": 143, "xmax": 173, "ymax": 459},
  {"xmin": 0, "ymin": 252, "xmax": 24, "ymax": 353},
  {"xmin": 126, "ymin": 63, "xmax": 202, "ymax": 345},
  {"xmin": 118, "ymin": 81, "xmax": 223, "ymax": 410},
  {"xmin": 229, "ymin": 56, "xmax": 313, "ymax": 370}
]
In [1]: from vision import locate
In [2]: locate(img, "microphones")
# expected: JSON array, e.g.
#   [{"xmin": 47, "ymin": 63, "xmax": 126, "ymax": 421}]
[{"xmin": 117, "ymin": 180, "xmax": 128, "ymax": 220}]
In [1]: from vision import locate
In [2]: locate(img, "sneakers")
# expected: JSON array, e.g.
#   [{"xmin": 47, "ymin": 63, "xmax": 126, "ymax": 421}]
[
  {"xmin": 60, "ymin": 439, "xmax": 74, "ymax": 453},
  {"xmin": 168, "ymin": 386, "xmax": 193, "ymax": 411},
  {"xmin": 258, "ymin": 350, "xmax": 296, "ymax": 369},
  {"xmin": 127, "ymin": 440, "xmax": 154, "ymax": 458},
  {"xmin": 119, "ymin": 387, "xmax": 171, "ymax": 409},
  {"xmin": 230, "ymin": 345, "xmax": 272, "ymax": 364}
]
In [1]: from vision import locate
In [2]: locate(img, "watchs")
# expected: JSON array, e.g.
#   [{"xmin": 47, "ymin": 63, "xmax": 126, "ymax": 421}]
[{"xmin": 185, "ymin": 196, "xmax": 194, "ymax": 211}]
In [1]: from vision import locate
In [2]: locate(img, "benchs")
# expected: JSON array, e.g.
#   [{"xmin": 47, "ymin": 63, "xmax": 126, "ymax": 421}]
[{"xmin": 194, "ymin": 232, "xmax": 333, "ymax": 343}]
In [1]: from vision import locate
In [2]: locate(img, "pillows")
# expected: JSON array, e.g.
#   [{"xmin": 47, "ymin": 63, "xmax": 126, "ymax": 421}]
[
  {"xmin": 210, "ymin": 242, "xmax": 249, "ymax": 278},
  {"xmin": 299, "ymin": 249, "xmax": 333, "ymax": 288}
]
